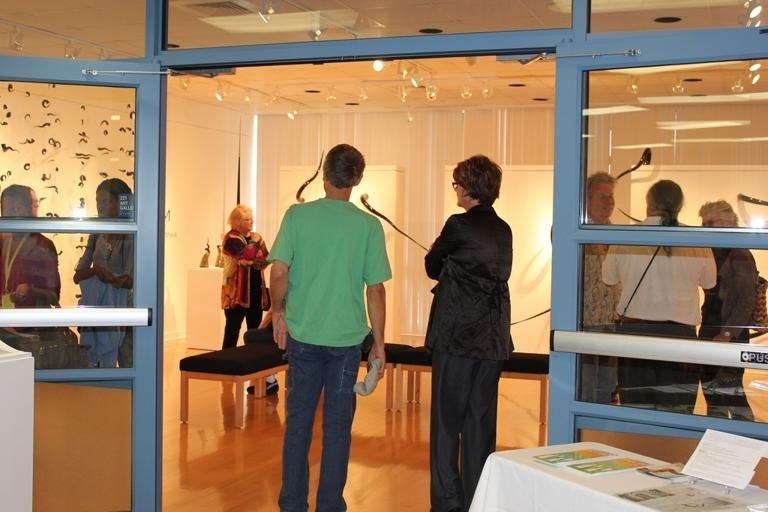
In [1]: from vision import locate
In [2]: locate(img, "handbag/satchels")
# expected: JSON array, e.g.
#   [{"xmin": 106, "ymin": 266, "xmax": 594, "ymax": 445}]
[
  {"xmin": 751, "ymin": 275, "xmax": 767, "ymax": 333},
  {"xmin": 16, "ymin": 289, "xmax": 92, "ymax": 369},
  {"xmin": 77, "ymin": 231, "xmax": 97, "ymax": 333}
]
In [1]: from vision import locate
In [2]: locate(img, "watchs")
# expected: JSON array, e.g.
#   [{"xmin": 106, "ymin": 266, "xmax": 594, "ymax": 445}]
[{"xmin": 720, "ymin": 332, "xmax": 733, "ymax": 337}]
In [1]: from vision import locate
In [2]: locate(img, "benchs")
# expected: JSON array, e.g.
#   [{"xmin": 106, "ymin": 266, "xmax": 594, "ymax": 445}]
[{"xmin": 395, "ymin": 347, "xmax": 549, "ymax": 443}]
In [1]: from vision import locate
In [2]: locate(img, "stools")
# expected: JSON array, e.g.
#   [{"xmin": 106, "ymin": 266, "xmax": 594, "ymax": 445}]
[
  {"xmin": 356, "ymin": 341, "xmax": 414, "ymax": 414},
  {"xmin": 177, "ymin": 340, "xmax": 288, "ymax": 431}
]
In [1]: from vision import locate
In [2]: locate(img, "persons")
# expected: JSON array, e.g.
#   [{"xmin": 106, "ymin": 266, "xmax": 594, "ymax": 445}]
[
  {"xmin": 697, "ymin": 202, "xmax": 758, "ymax": 422},
  {"xmin": 221, "ymin": 205, "xmax": 271, "ymax": 349},
  {"xmin": 268, "ymin": 143, "xmax": 392, "ymax": 511},
  {"xmin": 244, "ymin": 287, "xmax": 280, "ymax": 398},
  {"xmin": 551, "ymin": 174, "xmax": 621, "ymax": 404},
  {"xmin": 602, "ymin": 179, "xmax": 716, "ymax": 413},
  {"xmin": 0, "ymin": 186, "xmax": 60, "ymax": 335},
  {"xmin": 424, "ymin": 156, "xmax": 515, "ymax": 512},
  {"xmin": 72, "ymin": 179, "xmax": 136, "ymax": 368}
]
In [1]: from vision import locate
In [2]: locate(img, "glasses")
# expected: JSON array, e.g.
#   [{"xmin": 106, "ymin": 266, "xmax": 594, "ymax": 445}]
[
  {"xmin": 701, "ymin": 218, "xmax": 723, "ymax": 228},
  {"xmin": 451, "ymin": 181, "xmax": 461, "ymax": 188}
]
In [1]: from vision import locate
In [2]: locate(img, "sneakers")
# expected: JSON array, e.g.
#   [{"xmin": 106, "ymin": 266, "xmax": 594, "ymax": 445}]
[{"xmin": 247, "ymin": 379, "xmax": 279, "ymax": 394}]
